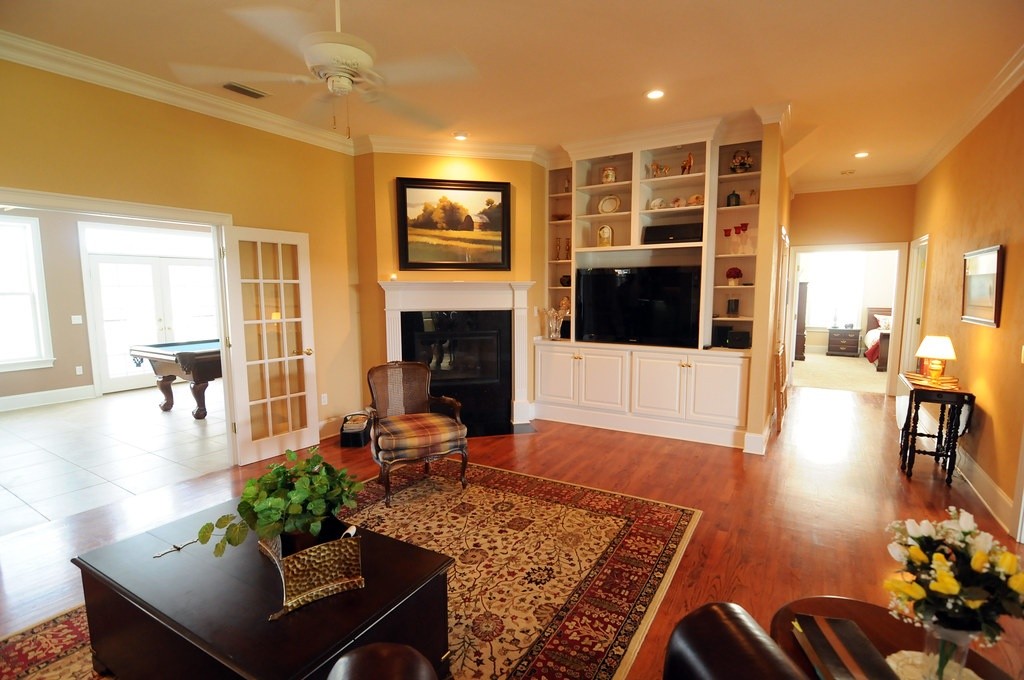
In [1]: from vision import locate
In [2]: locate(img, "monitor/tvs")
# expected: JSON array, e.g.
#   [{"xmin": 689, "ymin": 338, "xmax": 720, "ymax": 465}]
[{"xmin": 576, "ymin": 266, "xmax": 699, "ymax": 349}]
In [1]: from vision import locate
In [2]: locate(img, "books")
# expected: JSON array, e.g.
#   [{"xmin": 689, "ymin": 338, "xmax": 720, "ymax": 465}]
[
  {"xmin": 791, "ymin": 612, "xmax": 900, "ymax": 680},
  {"xmin": 344, "ymin": 414, "xmax": 368, "ymax": 433}
]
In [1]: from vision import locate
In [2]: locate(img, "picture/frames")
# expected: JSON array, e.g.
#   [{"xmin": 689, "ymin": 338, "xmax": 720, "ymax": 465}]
[
  {"xmin": 960, "ymin": 244, "xmax": 1004, "ymax": 328},
  {"xmin": 396, "ymin": 176, "xmax": 512, "ymax": 273}
]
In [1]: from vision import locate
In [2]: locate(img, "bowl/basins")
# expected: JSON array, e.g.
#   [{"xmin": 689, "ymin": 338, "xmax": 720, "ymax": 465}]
[{"xmin": 552, "ymin": 214, "xmax": 570, "ymax": 220}]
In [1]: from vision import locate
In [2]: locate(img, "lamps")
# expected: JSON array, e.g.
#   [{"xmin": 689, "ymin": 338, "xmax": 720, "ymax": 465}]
[
  {"xmin": 913, "ymin": 335, "xmax": 957, "ymax": 386},
  {"xmin": 320, "ymin": 67, "xmax": 359, "ymax": 141}
]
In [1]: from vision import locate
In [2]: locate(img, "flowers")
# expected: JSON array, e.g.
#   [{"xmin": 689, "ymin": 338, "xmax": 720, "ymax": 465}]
[{"xmin": 879, "ymin": 505, "xmax": 1024, "ymax": 680}]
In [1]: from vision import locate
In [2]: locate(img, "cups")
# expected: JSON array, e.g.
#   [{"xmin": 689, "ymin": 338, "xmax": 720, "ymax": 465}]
[{"xmin": 727, "ymin": 298, "xmax": 739, "ymax": 317}]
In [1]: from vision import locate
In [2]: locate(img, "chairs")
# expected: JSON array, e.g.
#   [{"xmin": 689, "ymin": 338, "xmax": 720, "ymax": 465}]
[{"xmin": 366, "ymin": 360, "xmax": 468, "ymax": 506}]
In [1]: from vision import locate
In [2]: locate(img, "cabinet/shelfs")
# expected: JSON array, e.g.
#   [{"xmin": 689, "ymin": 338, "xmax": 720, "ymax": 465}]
[{"xmin": 530, "ymin": 109, "xmax": 777, "ymax": 454}]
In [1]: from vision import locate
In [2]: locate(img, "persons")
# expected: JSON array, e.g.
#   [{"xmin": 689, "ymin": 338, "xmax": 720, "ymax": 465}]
[{"xmin": 563, "ymin": 175, "xmax": 569, "ymax": 193}]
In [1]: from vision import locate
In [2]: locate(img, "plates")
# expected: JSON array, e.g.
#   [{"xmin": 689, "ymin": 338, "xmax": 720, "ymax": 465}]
[
  {"xmin": 688, "ymin": 194, "xmax": 703, "ymax": 206},
  {"xmin": 651, "ymin": 198, "xmax": 666, "ymax": 210},
  {"xmin": 597, "ymin": 195, "xmax": 620, "ymax": 214},
  {"xmin": 670, "ymin": 197, "xmax": 686, "ymax": 208}
]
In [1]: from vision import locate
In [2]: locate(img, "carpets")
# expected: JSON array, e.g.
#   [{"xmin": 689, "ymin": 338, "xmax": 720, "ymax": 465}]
[{"xmin": 0, "ymin": 459, "xmax": 704, "ymax": 680}]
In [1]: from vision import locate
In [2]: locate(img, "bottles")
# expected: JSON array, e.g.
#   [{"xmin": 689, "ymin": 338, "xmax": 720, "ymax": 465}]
[
  {"xmin": 560, "ymin": 274, "xmax": 571, "ymax": 287},
  {"xmin": 727, "ymin": 190, "xmax": 740, "ymax": 207},
  {"xmin": 600, "ymin": 166, "xmax": 617, "ymax": 183},
  {"xmin": 721, "ymin": 222, "xmax": 752, "ymax": 254},
  {"xmin": 564, "ymin": 175, "xmax": 569, "ymax": 192}
]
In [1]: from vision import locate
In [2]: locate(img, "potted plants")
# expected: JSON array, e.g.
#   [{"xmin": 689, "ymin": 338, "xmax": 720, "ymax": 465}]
[{"xmin": 152, "ymin": 446, "xmax": 365, "ymax": 553}]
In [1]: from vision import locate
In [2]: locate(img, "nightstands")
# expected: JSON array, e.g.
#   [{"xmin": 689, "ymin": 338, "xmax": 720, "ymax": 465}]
[{"xmin": 826, "ymin": 326, "xmax": 862, "ymax": 357}]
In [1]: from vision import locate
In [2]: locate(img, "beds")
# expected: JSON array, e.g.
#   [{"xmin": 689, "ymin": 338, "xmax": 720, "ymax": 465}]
[{"xmin": 863, "ymin": 307, "xmax": 892, "ymax": 372}]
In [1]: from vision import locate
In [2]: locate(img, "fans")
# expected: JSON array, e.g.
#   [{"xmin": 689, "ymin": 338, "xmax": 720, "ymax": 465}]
[{"xmin": 164, "ymin": 0, "xmax": 495, "ymax": 132}]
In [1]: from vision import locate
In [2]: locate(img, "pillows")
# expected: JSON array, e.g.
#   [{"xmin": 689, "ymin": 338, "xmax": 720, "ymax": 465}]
[{"xmin": 874, "ymin": 314, "xmax": 892, "ymax": 330}]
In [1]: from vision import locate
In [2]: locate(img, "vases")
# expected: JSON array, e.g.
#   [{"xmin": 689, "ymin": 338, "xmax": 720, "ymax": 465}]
[{"xmin": 920, "ymin": 617, "xmax": 972, "ymax": 680}]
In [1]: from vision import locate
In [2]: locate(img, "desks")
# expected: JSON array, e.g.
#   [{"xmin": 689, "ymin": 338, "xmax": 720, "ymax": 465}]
[
  {"xmin": 772, "ymin": 594, "xmax": 1014, "ymax": 680},
  {"xmin": 896, "ymin": 374, "xmax": 975, "ymax": 485},
  {"xmin": 129, "ymin": 338, "xmax": 223, "ymax": 419}
]
